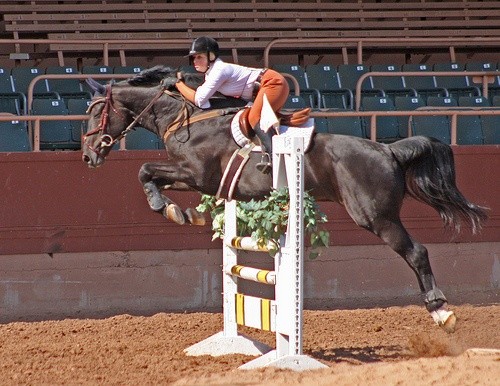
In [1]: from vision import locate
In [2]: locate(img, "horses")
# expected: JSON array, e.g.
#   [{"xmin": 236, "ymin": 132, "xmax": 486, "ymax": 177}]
[{"xmin": 80, "ymin": 64, "xmax": 494, "ymax": 338}]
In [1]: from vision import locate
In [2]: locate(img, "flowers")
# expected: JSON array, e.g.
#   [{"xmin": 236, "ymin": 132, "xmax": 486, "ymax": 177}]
[{"xmin": 197, "ymin": 186, "xmax": 331, "ymax": 258}]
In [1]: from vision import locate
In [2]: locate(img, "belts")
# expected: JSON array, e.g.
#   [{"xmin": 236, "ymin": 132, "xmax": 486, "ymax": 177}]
[{"xmin": 252, "ymin": 67, "xmax": 269, "ymax": 96}]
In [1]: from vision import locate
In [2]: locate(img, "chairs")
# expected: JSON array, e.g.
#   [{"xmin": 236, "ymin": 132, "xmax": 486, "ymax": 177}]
[{"xmin": 0, "ymin": 64, "xmax": 500, "ymax": 152}]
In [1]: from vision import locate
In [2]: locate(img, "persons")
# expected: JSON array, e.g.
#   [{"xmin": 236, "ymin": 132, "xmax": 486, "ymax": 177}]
[{"xmin": 160, "ymin": 36, "xmax": 290, "ymax": 173}]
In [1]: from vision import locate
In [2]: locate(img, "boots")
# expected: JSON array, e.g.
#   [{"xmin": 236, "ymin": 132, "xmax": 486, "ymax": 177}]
[{"xmin": 255, "ymin": 122, "xmax": 278, "ymax": 172}]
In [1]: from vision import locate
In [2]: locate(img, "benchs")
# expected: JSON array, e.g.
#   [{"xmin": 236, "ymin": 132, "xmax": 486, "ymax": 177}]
[{"xmin": 0, "ymin": 0, "xmax": 500, "ymax": 67}]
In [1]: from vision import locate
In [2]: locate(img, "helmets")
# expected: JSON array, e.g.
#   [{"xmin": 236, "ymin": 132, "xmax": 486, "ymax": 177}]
[{"xmin": 184, "ymin": 36, "xmax": 218, "ymax": 57}]
